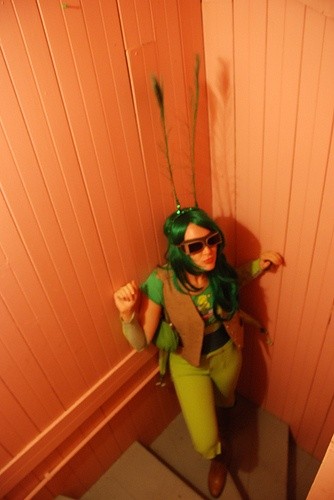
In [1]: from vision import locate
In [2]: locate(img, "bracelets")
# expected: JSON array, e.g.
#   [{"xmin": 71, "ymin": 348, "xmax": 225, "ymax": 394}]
[{"xmin": 119, "ymin": 312, "xmax": 135, "ymax": 324}]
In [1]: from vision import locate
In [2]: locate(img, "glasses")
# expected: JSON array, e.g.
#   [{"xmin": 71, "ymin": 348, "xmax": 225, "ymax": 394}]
[{"xmin": 178, "ymin": 233, "xmax": 222, "ymax": 255}]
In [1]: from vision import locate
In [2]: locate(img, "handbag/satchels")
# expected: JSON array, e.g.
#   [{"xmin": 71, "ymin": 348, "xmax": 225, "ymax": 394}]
[{"xmin": 154, "ymin": 320, "xmax": 180, "ymax": 351}]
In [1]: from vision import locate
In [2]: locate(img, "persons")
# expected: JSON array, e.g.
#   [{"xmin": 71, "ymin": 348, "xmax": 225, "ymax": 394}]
[{"xmin": 114, "ymin": 207, "xmax": 284, "ymax": 498}]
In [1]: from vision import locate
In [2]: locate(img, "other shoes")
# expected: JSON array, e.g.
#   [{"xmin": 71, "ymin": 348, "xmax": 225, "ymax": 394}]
[{"xmin": 208, "ymin": 449, "xmax": 227, "ymax": 496}]
